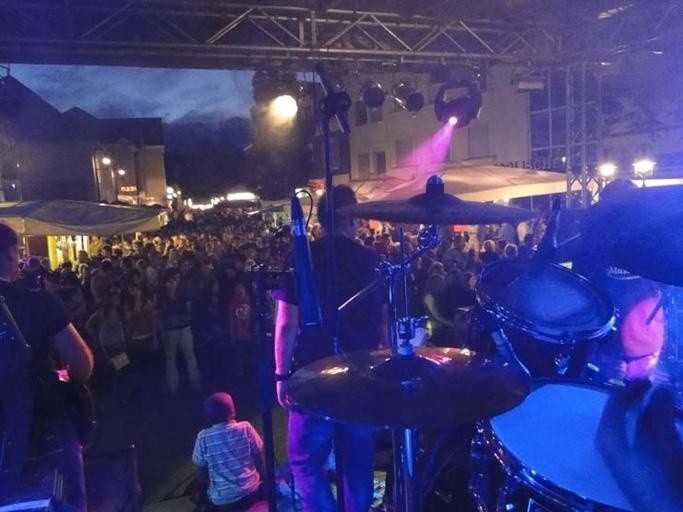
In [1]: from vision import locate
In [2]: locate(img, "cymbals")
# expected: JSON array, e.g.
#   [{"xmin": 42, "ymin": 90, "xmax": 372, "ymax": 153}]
[
  {"xmin": 334, "ymin": 189, "xmax": 542, "ymax": 225},
  {"xmin": 578, "ymin": 184, "xmax": 682, "ymax": 287},
  {"xmin": 280, "ymin": 344, "xmax": 531, "ymax": 432}
]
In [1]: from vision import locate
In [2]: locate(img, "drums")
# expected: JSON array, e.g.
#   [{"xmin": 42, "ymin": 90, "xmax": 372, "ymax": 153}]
[
  {"xmin": 464, "ymin": 253, "xmax": 622, "ymax": 385},
  {"xmin": 468, "ymin": 377, "xmax": 683, "ymax": 510}
]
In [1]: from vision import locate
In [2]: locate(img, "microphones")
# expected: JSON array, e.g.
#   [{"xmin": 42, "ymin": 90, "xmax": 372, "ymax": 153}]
[
  {"xmin": 0, "ymin": 293, "xmax": 31, "ymax": 355},
  {"xmin": 288, "ymin": 196, "xmax": 326, "ymax": 332},
  {"xmin": 525, "ymin": 197, "xmax": 561, "ymax": 277},
  {"xmin": 26, "ymin": 256, "xmax": 53, "ymax": 275},
  {"xmin": 312, "ymin": 60, "xmax": 354, "ymax": 140}
]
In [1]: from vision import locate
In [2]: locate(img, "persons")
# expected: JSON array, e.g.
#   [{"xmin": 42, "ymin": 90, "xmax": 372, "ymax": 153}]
[{"xmin": 567, "ymin": 177, "xmax": 665, "ymax": 383}]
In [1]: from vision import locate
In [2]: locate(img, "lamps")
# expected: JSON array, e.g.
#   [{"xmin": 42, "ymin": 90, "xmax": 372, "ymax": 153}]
[{"xmin": 315, "ymin": 59, "xmax": 484, "ymax": 130}]
